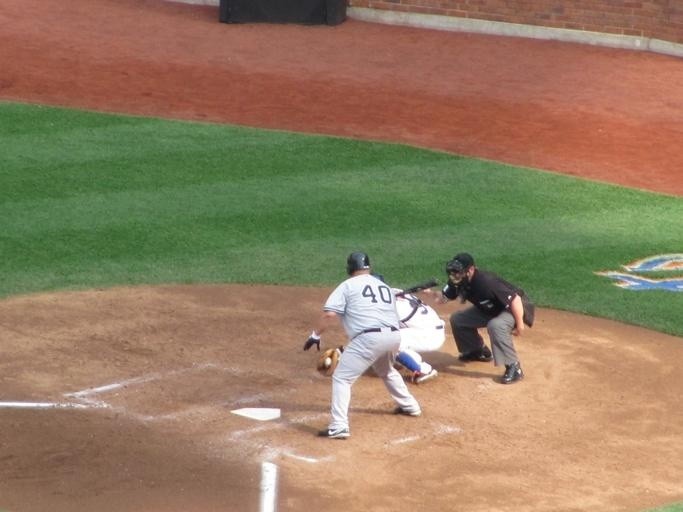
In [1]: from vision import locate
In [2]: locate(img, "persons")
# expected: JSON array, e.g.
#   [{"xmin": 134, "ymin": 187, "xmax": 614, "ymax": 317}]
[
  {"xmin": 421, "ymin": 254, "xmax": 535, "ymax": 384},
  {"xmin": 370, "ymin": 272, "xmax": 446, "ymax": 385},
  {"xmin": 300, "ymin": 251, "xmax": 421, "ymax": 438}
]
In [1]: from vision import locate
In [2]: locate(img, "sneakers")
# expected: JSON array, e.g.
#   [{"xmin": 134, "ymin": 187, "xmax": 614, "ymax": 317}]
[
  {"xmin": 318, "ymin": 429, "xmax": 351, "ymax": 439},
  {"xmin": 394, "ymin": 407, "xmax": 421, "ymax": 416},
  {"xmin": 415, "ymin": 366, "xmax": 438, "ymax": 385},
  {"xmin": 459, "ymin": 346, "xmax": 493, "ymax": 361},
  {"xmin": 501, "ymin": 363, "xmax": 523, "ymax": 384}
]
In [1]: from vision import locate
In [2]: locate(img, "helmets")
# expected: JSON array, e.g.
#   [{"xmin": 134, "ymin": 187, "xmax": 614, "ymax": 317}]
[
  {"xmin": 347, "ymin": 252, "xmax": 371, "ymax": 274},
  {"xmin": 371, "ymin": 274, "xmax": 385, "ymax": 282},
  {"xmin": 447, "ymin": 252, "xmax": 474, "ymax": 303}
]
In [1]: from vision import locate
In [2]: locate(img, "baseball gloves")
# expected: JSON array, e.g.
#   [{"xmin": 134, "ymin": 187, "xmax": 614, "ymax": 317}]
[{"xmin": 317, "ymin": 348, "xmax": 339, "ymax": 376}]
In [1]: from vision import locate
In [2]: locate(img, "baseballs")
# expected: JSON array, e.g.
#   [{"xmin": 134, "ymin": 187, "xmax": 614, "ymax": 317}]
[
  {"xmin": 325, "ymin": 357, "xmax": 332, "ymax": 368},
  {"xmin": 423, "ymin": 288, "xmax": 431, "ymax": 293}
]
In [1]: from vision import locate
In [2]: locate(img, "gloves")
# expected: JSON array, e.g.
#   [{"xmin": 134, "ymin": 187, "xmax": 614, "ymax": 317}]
[{"xmin": 303, "ymin": 330, "xmax": 320, "ymax": 351}]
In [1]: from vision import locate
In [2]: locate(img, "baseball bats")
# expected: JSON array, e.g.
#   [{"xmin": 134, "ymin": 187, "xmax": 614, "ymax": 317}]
[{"xmin": 395, "ymin": 278, "xmax": 438, "ymax": 297}]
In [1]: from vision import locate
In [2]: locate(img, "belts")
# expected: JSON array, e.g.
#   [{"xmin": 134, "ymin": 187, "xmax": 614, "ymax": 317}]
[
  {"xmin": 351, "ymin": 327, "xmax": 397, "ymax": 339},
  {"xmin": 436, "ymin": 325, "xmax": 445, "ymax": 329}
]
